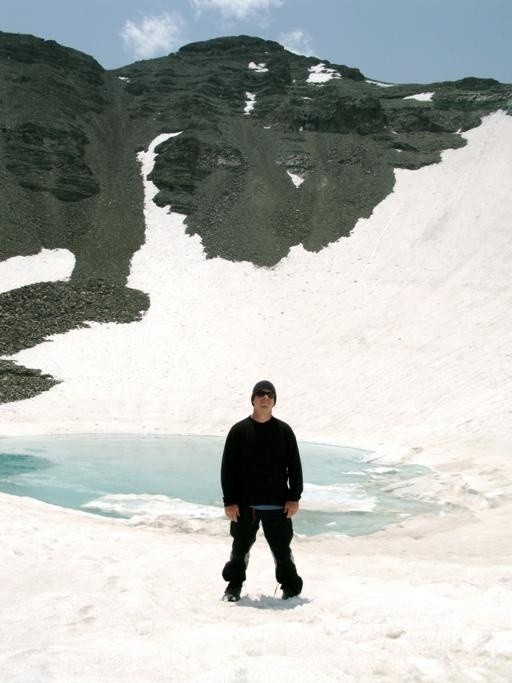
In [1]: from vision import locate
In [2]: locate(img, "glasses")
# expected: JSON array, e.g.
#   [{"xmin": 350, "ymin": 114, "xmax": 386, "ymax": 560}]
[{"xmin": 255, "ymin": 391, "xmax": 275, "ymax": 399}]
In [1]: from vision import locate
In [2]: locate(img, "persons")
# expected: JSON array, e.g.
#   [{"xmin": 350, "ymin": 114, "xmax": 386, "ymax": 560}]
[{"xmin": 219, "ymin": 380, "xmax": 304, "ymax": 602}]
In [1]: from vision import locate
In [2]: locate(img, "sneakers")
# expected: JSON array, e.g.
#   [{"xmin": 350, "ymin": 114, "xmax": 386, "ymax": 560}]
[{"xmin": 222, "ymin": 580, "xmax": 243, "ymax": 601}]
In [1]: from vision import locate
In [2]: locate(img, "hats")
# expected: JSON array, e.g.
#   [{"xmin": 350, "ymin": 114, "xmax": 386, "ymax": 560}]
[{"xmin": 251, "ymin": 379, "xmax": 276, "ymax": 407}]
